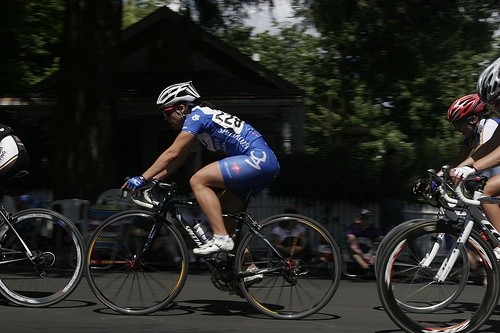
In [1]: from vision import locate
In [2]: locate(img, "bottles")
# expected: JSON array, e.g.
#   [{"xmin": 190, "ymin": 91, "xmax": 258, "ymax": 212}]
[{"xmin": 191, "ymin": 219, "xmax": 212, "ymax": 243}]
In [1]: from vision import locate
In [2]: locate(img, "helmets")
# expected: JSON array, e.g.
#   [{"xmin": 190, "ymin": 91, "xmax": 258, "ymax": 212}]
[
  {"xmin": 156, "ymin": 80, "xmax": 200, "ymax": 109},
  {"xmin": 446, "ymin": 93, "xmax": 484, "ymax": 123},
  {"xmin": 476, "ymin": 56, "xmax": 500, "ymax": 104}
]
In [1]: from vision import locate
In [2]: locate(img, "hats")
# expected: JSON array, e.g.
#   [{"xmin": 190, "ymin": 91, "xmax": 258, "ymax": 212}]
[{"xmin": 360, "ymin": 209, "xmax": 373, "ymax": 217}]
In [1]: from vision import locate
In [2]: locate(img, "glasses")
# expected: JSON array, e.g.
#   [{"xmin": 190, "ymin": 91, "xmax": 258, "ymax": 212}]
[
  {"xmin": 495, "ymin": 106, "xmax": 500, "ymax": 113},
  {"xmin": 453, "ymin": 113, "xmax": 475, "ymax": 128},
  {"xmin": 162, "ymin": 107, "xmax": 178, "ymax": 118}
]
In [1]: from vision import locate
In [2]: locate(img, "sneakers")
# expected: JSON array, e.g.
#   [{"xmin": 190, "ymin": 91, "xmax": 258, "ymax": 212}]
[
  {"xmin": 244, "ymin": 265, "xmax": 264, "ymax": 286},
  {"xmin": 192, "ymin": 234, "xmax": 235, "ymax": 254}
]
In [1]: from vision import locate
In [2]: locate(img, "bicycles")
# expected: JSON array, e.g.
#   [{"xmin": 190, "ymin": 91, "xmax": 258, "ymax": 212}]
[
  {"xmin": 0, "ymin": 166, "xmax": 88, "ymax": 308},
  {"xmin": 376, "ymin": 163, "xmax": 500, "ymax": 333},
  {"xmin": 372, "ymin": 178, "xmax": 500, "ymax": 315},
  {"xmin": 82, "ymin": 175, "xmax": 343, "ymax": 320}
]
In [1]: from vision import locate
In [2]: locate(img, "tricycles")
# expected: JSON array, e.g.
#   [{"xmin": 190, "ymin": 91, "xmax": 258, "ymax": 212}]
[{"xmin": 311, "ymin": 236, "xmax": 449, "ymax": 284}]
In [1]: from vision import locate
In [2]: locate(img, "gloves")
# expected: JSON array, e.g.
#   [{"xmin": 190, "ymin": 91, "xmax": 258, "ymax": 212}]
[{"xmin": 453, "ymin": 166, "xmax": 476, "ymax": 179}]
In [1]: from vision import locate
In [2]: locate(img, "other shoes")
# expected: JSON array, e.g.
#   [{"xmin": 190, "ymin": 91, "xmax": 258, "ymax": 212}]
[{"xmin": 478, "ymin": 247, "xmax": 500, "ymax": 261}]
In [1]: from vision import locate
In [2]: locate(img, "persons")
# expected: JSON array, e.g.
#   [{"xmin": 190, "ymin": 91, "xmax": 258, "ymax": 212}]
[
  {"xmin": 5, "ymin": 195, "xmax": 77, "ymax": 269},
  {"xmin": 343, "ymin": 207, "xmax": 389, "ymax": 282},
  {"xmin": 0, "ymin": 123, "xmax": 30, "ymax": 179},
  {"xmin": 427, "ymin": 56, "xmax": 500, "ymax": 287},
  {"xmin": 175, "ymin": 192, "xmax": 213, "ymax": 271},
  {"xmin": 128, "ymin": 203, "xmax": 184, "ymax": 272},
  {"xmin": 121, "ymin": 81, "xmax": 280, "ymax": 295},
  {"xmin": 273, "ymin": 208, "xmax": 314, "ymax": 279}
]
